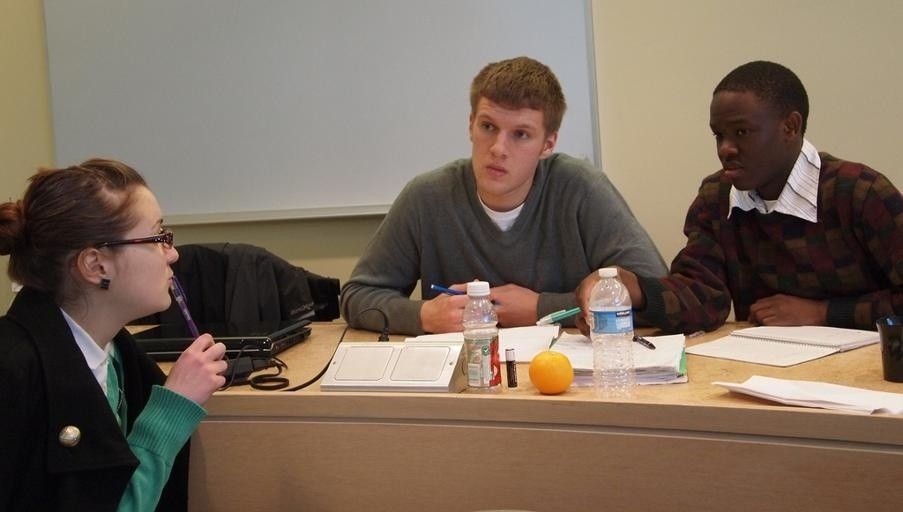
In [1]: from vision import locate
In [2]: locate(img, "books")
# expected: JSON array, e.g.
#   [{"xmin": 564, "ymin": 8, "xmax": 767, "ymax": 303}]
[{"xmin": 683, "ymin": 323, "xmax": 881, "ymax": 368}]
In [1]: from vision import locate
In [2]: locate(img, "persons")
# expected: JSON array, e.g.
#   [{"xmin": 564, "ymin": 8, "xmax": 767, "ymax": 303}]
[
  {"xmin": 578, "ymin": 60, "xmax": 903, "ymax": 336},
  {"xmin": 340, "ymin": 56, "xmax": 671, "ymax": 337},
  {"xmin": 0, "ymin": 158, "xmax": 229, "ymax": 512}
]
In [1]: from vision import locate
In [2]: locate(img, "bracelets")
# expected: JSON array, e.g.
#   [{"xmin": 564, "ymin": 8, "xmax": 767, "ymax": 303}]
[{"xmin": 826, "ymin": 300, "xmax": 856, "ymax": 327}]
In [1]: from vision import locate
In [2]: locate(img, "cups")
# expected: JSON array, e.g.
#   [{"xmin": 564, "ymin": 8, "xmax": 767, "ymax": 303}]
[{"xmin": 875, "ymin": 316, "xmax": 902, "ymax": 383}]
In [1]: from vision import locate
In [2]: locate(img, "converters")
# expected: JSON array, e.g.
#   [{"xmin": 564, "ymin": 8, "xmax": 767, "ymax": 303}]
[{"xmin": 220, "ymin": 356, "xmax": 254, "ymax": 382}]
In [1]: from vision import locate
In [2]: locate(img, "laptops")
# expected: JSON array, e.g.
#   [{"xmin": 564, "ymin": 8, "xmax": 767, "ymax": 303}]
[{"xmin": 130, "ymin": 316, "xmax": 315, "ymax": 360}]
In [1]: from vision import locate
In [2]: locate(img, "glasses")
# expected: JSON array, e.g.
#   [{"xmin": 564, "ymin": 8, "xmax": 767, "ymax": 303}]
[{"xmin": 93, "ymin": 231, "xmax": 173, "ymax": 249}]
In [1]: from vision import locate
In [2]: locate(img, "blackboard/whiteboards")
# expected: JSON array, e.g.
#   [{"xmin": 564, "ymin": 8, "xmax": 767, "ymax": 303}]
[{"xmin": 42, "ymin": 0, "xmax": 603, "ymax": 225}]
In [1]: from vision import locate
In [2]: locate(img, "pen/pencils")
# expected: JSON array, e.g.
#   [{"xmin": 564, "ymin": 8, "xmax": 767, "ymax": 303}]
[
  {"xmin": 430, "ymin": 284, "xmax": 499, "ymax": 304},
  {"xmin": 170, "ymin": 276, "xmax": 200, "ymax": 339},
  {"xmin": 633, "ymin": 335, "xmax": 655, "ymax": 349},
  {"xmin": 536, "ymin": 306, "xmax": 581, "ymax": 325}
]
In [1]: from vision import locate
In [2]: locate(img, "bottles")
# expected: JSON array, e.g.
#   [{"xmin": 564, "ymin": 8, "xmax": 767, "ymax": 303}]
[
  {"xmin": 587, "ymin": 267, "xmax": 636, "ymax": 395},
  {"xmin": 462, "ymin": 281, "xmax": 502, "ymax": 395}
]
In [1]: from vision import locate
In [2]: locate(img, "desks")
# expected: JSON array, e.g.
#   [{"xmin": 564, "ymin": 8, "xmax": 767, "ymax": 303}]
[{"xmin": 130, "ymin": 323, "xmax": 901, "ymax": 512}]
